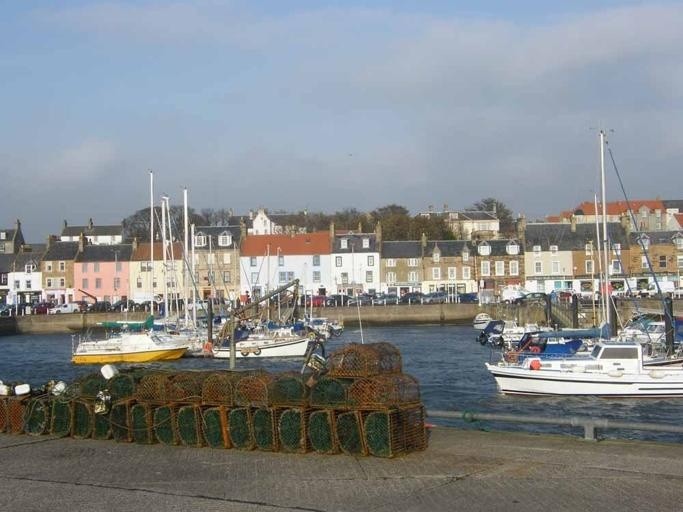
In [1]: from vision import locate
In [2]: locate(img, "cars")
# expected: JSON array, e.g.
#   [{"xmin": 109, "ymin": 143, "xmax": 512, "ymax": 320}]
[
  {"xmin": 265, "ymin": 281, "xmax": 683, "ymax": 308},
  {"xmin": 0, "ymin": 296, "xmax": 231, "ymax": 316}
]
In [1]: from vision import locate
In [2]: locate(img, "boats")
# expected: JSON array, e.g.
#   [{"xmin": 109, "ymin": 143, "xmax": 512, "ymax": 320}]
[
  {"xmin": 71, "ymin": 324, "xmax": 190, "ymax": 366},
  {"xmin": 483, "ymin": 337, "xmax": 682, "ymax": 398}
]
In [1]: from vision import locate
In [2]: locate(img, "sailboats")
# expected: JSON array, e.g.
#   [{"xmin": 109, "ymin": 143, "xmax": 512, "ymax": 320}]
[
  {"xmin": 473, "ymin": 126, "xmax": 682, "ymax": 364},
  {"xmin": 104, "ymin": 168, "xmax": 344, "ymax": 360}
]
